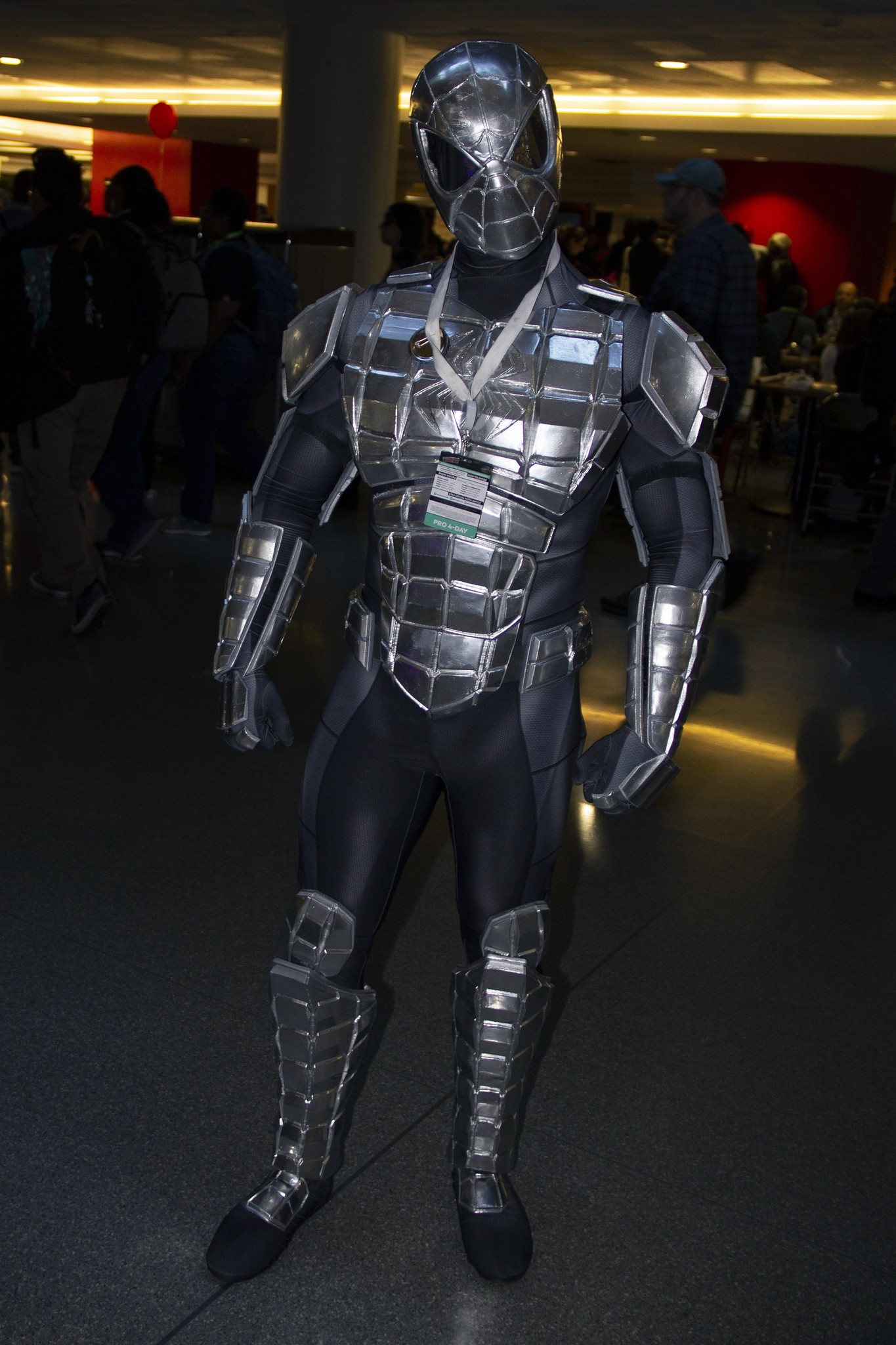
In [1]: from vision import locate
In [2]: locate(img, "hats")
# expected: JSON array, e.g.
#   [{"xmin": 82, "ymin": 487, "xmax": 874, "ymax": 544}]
[
  {"xmin": 653, "ymin": 158, "xmax": 726, "ymax": 200},
  {"xmin": 768, "ymin": 231, "xmax": 792, "ymax": 250}
]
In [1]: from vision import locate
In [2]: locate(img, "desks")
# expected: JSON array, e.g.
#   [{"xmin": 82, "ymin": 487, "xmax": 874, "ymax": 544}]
[{"xmin": 730, "ymin": 376, "xmax": 837, "ymax": 526}]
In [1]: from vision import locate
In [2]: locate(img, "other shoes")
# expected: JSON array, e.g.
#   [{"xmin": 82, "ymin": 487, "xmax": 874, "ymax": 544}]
[
  {"xmin": 98, "ymin": 538, "xmax": 144, "ymax": 562},
  {"xmin": 447, "ymin": 1135, "xmax": 533, "ymax": 1284},
  {"xmin": 30, "ymin": 569, "xmax": 73, "ymax": 597},
  {"xmin": 203, "ymin": 1168, "xmax": 336, "ymax": 1282},
  {"xmin": 118, "ymin": 513, "xmax": 162, "ymax": 560},
  {"xmin": 157, "ymin": 515, "xmax": 213, "ymax": 536},
  {"xmin": 600, "ymin": 581, "xmax": 643, "ymax": 616},
  {"xmin": 70, "ymin": 582, "xmax": 118, "ymax": 633}
]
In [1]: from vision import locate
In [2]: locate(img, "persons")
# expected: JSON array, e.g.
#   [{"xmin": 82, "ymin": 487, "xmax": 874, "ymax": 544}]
[
  {"xmin": 0, "ymin": 148, "xmax": 299, "ymax": 637},
  {"xmin": 380, "ymin": 201, "xmax": 456, "ymax": 274},
  {"xmin": 204, "ymin": 38, "xmax": 733, "ymax": 1283},
  {"xmin": 556, "ymin": 158, "xmax": 896, "ymax": 617}
]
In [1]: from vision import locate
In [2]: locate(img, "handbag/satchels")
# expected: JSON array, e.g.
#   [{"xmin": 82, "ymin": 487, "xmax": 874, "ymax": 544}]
[{"xmin": 618, "ymin": 245, "xmax": 632, "ymax": 291}]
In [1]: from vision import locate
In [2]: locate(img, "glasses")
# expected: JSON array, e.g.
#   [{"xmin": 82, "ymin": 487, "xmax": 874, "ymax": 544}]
[{"xmin": 666, "ymin": 182, "xmax": 692, "ymax": 192}]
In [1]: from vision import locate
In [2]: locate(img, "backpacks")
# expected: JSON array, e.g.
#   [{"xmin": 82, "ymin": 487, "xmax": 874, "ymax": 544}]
[
  {"xmin": 204, "ymin": 232, "xmax": 298, "ymax": 359},
  {"xmin": 124, "ymin": 221, "xmax": 211, "ymax": 354}
]
